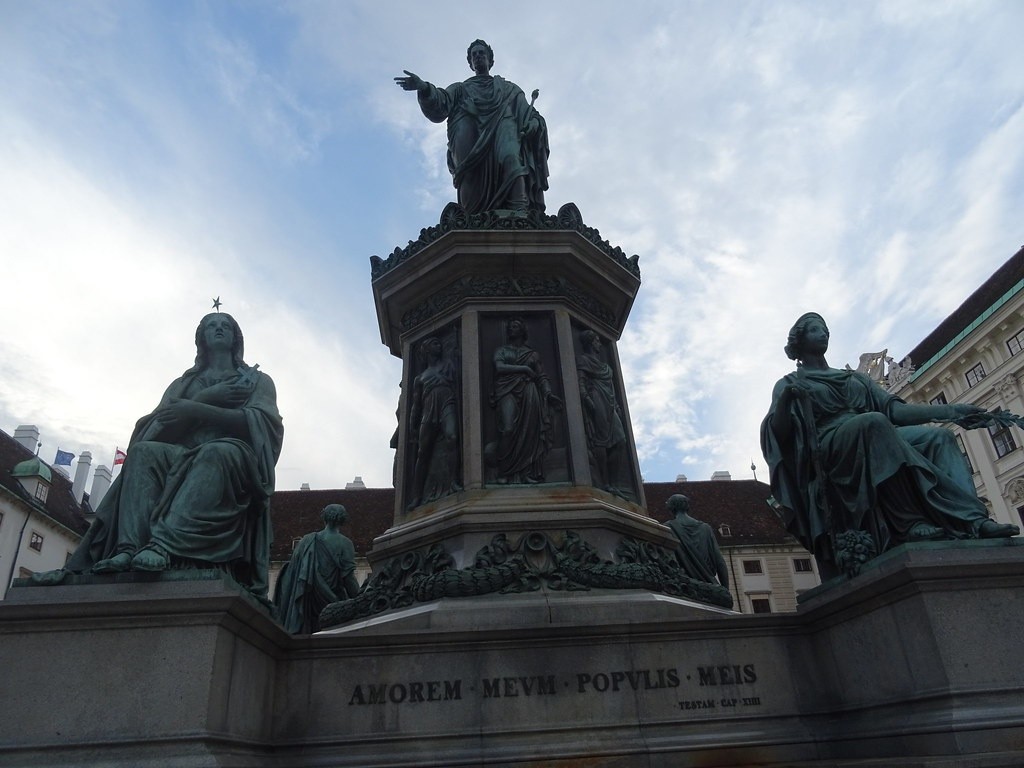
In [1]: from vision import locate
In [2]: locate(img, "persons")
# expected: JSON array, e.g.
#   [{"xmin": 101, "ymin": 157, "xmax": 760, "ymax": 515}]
[
  {"xmin": 761, "ymin": 313, "xmax": 1024, "ymax": 553},
  {"xmin": 293, "ymin": 503, "xmax": 354, "ymax": 634},
  {"xmin": 408, "ymin": 337, "xmax": 463, "ymax": 511},
  {"xmin": 93, "ymin": 313, "xmax": 276, "ymax": 573},
  {"xmin": 485, "ymin": 317, "xmax": 563, "ymax": 484},
  {"xmin": 575, "ymin": 330, "xmax": 630, "ymax": 501},
  {"xmin": 663, "ymin": 494, "xmax": 728, "ymax": 592},
  {"xmin": 394, "ymin": 39, "xmax": 545, "ymax": 212}
]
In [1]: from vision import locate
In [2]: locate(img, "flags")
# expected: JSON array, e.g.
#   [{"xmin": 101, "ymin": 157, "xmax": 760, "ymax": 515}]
[
  {"xmin": 54, "ymin": 450, "xmax": 75, "ymax": 466},
  {"xmin": 114, "ymin": 450, "xmax": 126, "ymax": 465}
]
